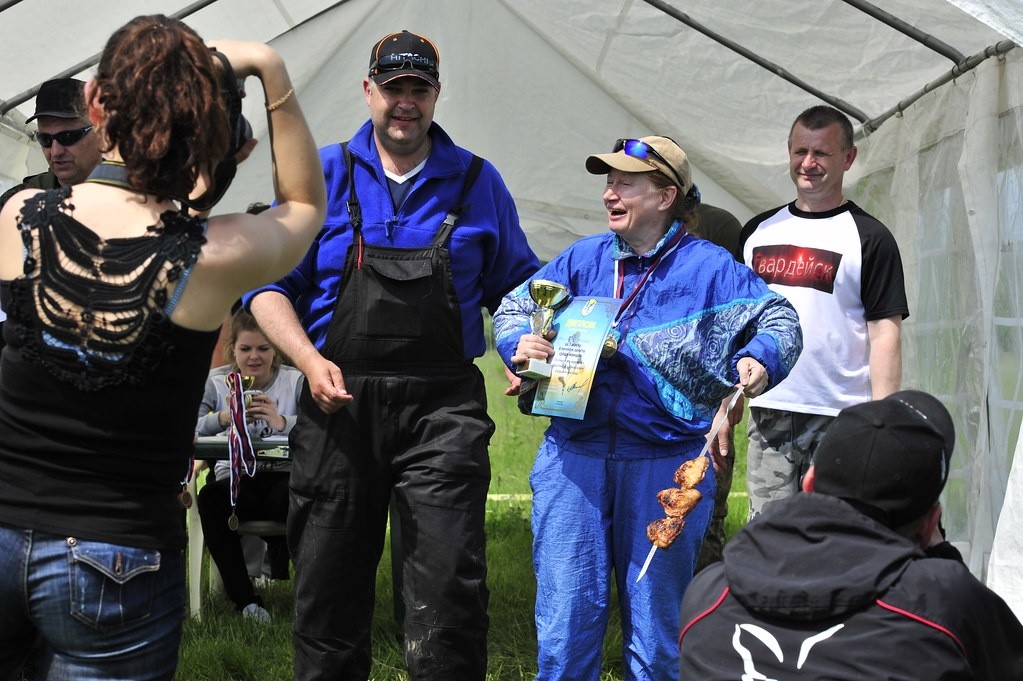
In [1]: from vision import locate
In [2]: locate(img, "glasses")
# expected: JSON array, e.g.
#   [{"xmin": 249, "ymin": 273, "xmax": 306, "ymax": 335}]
[
  {"xmin": 35, "ymin": 123, "xmax": 95, "ymax": 148},
  {"xmin": 613, "ymin": 138, "xmax": 685, "ymax": 186},
  {"xmin": 369, "ymin": 53, "xmax": 440, "ymax": 75}
]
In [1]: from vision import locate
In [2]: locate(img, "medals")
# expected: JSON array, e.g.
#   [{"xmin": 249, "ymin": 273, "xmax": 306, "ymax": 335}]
[
  {"xmin": 226, "ymin": 372, "xmax": 264, "ymax": 531},
  {"xmin": 600, "ymin": 224, "xmax": 686, "ymax": 358}
]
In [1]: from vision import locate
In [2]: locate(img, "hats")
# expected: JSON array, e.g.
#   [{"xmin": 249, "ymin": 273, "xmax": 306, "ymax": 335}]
[
  {"xmin": 586, "ymin": 134, "xmax": 693, "ymax": 196},
  {"xmin": 813, "ymin": 389, "xmax": 956, "ymax": 529},
  {"xmin": 25, "ymin": 76, "xmax": 89, "ymax": 123},
  {"xmin": 368, "ymin": 29, "xmax": 439, "ymax": 90}
]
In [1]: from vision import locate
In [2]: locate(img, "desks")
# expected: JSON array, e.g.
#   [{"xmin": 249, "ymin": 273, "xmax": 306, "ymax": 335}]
[{"xmin": 185, "ymin": 434, "xmax": 408, "ymax": 622}]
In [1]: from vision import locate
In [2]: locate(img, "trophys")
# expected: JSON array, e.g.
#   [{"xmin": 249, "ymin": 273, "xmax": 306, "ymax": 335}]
[{"xmin": 515, "ymin": 279, "xmax": 570, "ymax": 381}]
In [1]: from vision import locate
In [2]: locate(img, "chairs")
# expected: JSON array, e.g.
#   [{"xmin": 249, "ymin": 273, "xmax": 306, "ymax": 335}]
[{"xmin": 185, "ymin": 459, "xmax": 287, "ymax": 622}]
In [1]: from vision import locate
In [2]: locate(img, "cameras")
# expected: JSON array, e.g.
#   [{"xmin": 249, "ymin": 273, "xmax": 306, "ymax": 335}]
[{"xmin": 219, "ymin": 88, "xmax": 254, "ymax": 154}]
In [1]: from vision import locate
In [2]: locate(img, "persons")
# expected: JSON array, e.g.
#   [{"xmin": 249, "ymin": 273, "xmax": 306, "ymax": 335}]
[
  {"xmin": 732, "ymin": 105, "xmax": 911, "ymax": 525},
  {"xmin": 0, "ymin": 13, "xmax": 330, "ymax": 681},
  {"xmin": 0, "ymin": 78, "xmax": 106, "ymax": 213},
  {"xmin": 197, "ymin": 305, "xmax": 306, "ymax": 625},
  {"xmin": 677, "ymin": 389, "xmax": 1023, "ymax": 681},
  {"xmin": 493, "ymin": 135, "xmax": 803, "ymax": 681},
  {"xmin": 240, "ymin": 30, "xmax": 544, "ymax": 681},
  {"xmin": 683, "ymin": 203, "xmax": 744, "ymax": 577},
  {"xmin": 232, "ymin": 202, "xmax": 271, "ymax": 578}
]
